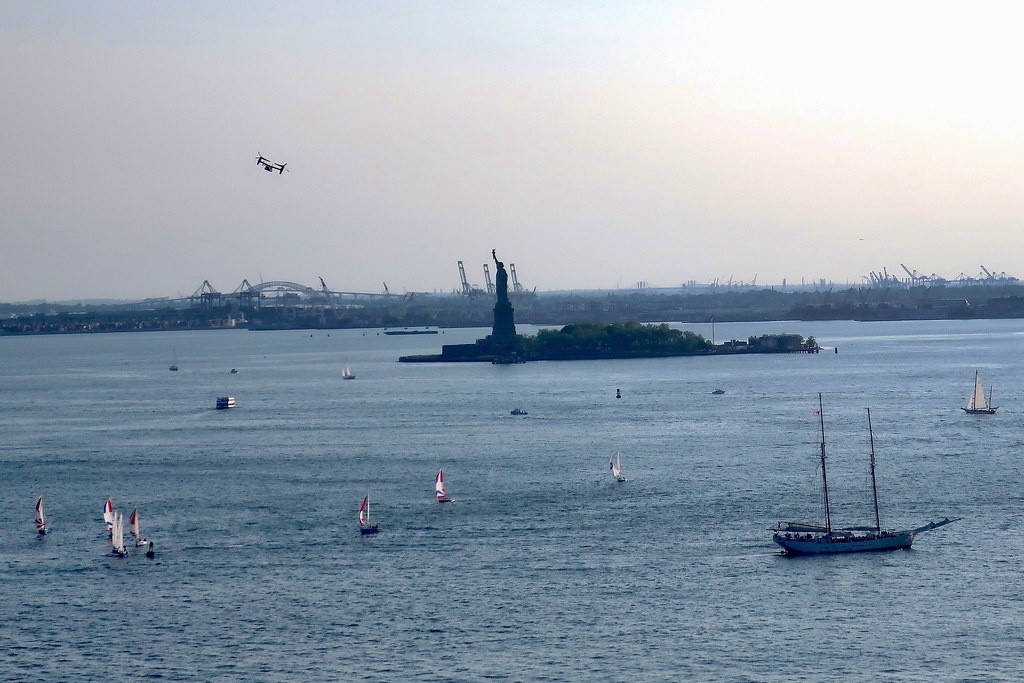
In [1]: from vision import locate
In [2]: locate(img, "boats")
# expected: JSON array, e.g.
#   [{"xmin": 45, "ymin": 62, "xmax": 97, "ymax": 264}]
[
  {"xmin": 231, "ymin": 369, "xmax": 239, "ymax": 373},
  {"xmin": 511, "ymin": 407, "xmax": 527, "ymax": 415},
  {"xmin": 712, "ymin": 387, "xmax": 725, "ymax": 394},
  {"xmin": 384, "ymin": 330, "xmax": 438, "ymax": 335}
]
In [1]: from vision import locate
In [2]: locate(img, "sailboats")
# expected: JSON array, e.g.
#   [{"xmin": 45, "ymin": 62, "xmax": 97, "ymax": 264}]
[
  {"xmin": 358, "ymin": 491, "xmax": 378, "ymax": 534},
  {"xmin": 34, "ymin": 496, "xmax": 48, "ymax": 534},
  {"xmin": 112, "ymin": 512, "xmax": 126, "ymax": 557},
  {"xmin": 609, "ymin": 451, "xmax": 627, "ymax": 482},
  {"xmin": 169, "ymin": 345, "xmax": 178, "ymax": 371},
  {"xmin": 342, "ymin": 367, "xmax": 356, "ymax": 378},
  {"xmin": 766, "ymin": 392, "xmax": 964, "ymax": 555},
  {"xmin": 129, "ymin": 505, "xmax": 148, "ymax": 546},
  {"xmin": 961, "ymin": 369, "xmax": 999, "ymax": 414},
  {"xmin": 435, "ymin": 469, "xmax": 453, "ymax": 502},
  {"xmin": 103, "ymin": 499, "xmax": 114, "ymax": 541}
]
